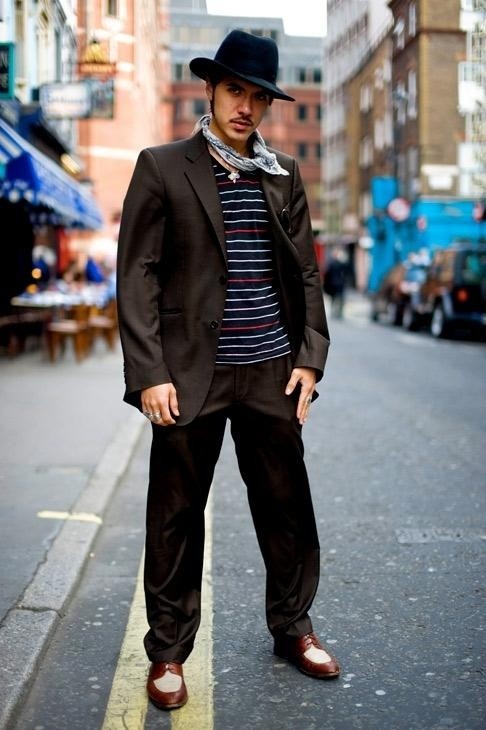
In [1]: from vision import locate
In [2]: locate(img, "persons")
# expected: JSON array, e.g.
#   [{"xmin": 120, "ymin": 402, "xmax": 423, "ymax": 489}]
[
  {"xmin": 57, "ymin": 236, "xmax": 107, "ymax": 289},
  {"xmin": 117, "ymin": 29, "xmax": 341, "ymax": 710},
  {"xmin": 322, "ymin": 252, "xmax": 346, "ymax": 318}
]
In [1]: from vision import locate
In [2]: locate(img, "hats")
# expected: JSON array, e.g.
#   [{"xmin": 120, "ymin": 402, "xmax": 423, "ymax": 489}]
[{"xmin": 188, "ymin": 30, "xmax": 296, "ymax": 101}]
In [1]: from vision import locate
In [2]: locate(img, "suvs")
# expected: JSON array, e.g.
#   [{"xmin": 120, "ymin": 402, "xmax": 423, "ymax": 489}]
[{"xmin": 399, "ymin": 238, "xmax": 486, "ymax": 340}]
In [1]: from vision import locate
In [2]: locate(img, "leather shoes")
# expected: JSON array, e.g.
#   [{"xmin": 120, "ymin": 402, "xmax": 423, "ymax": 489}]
[
  {"xmin": 146, "ymin": 661, "xmax": 187, "ymax": 708},
  {"xmin": 273, "ymin": 632, "xmax": 339, "ymax": 678}
]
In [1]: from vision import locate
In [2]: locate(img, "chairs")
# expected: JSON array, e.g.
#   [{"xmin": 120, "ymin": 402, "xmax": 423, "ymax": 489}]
[{"xmin": 12, "ymin": 290, "xmax": 117, "ymax": 363}]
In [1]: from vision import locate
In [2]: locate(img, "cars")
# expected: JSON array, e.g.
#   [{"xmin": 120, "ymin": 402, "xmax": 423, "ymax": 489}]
[{"xmin": 373, "ymin": 258, "xmax": 430, "ymax": 330}]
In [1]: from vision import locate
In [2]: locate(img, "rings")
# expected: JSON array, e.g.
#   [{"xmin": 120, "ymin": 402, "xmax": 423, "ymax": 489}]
[
  {"xmin": 154, "ymin": 413, "xmax": 160, "ymax": 420},
  {"xmin": 147, "ymin": 413, "xmax": 153, "ymax": 419}
]
